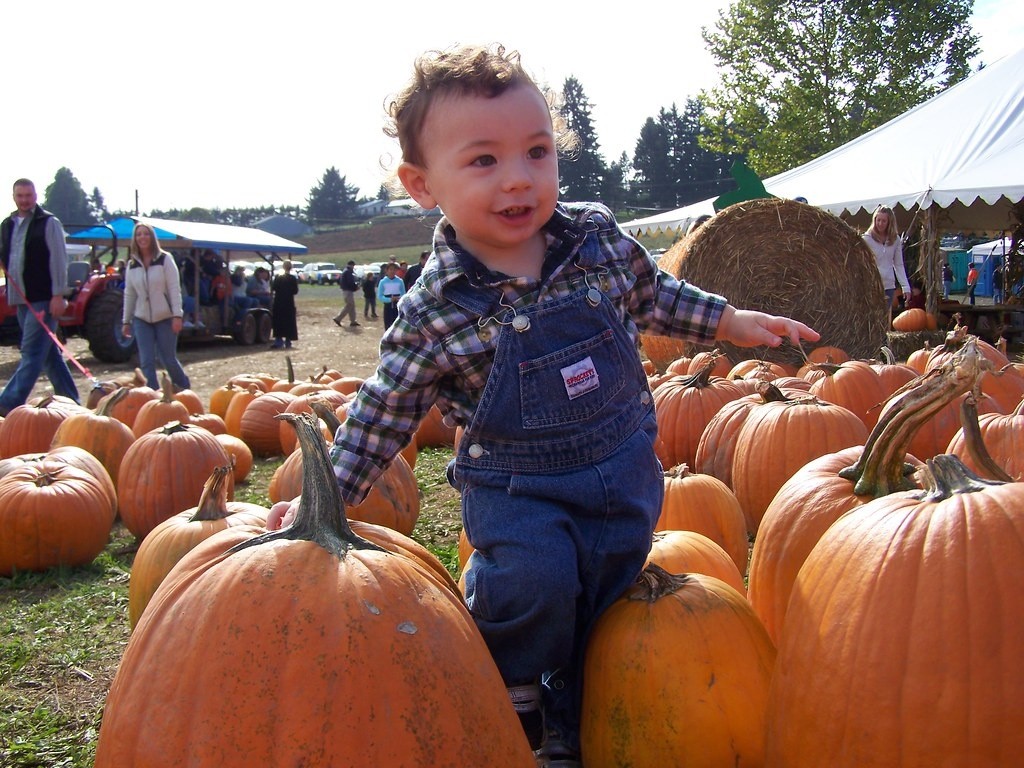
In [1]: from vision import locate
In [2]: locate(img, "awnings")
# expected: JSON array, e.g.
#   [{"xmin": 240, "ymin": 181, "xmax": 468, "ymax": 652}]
[{"xmin": 972, "ymin": 237, "xmax": 1024, "ymax": 297}]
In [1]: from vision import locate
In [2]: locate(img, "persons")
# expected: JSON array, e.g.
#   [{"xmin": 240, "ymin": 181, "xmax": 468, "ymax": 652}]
[
  {"xmin": 265, "ymin": 45, "xmax": 821, "ymax": 768},
  {"xmin": 861, "ymin": 205, "xmax": 911, "ymax": 309},
  {"xmin": 363, "ymin": 272, "xmax": 379, "ymax": 318},
  {"xmin": 333, "ymin": 260, "xmax": 361, "ymax": 327},
  {"xmin": 116, "ymin": 248, "xmax": 299, "ymax": 349},
  {"xmin": 991, "ymin": 264, "xmax": 1004, "ymax": 305},
  {"xmin": 942, "ymin": 263, "xmax": 953, "ymax": 299},
  {"xmin": 377, "ymin": 250, "xmax": 431, "ymax": 331},
  {"xmin": 122, "ymin": 222, "xmax": 190, "ymax": 391},
  {"xmin": 0, "ymin": 179, "xmax": 81, "ymax": 419},
  {"xmin": 967, "ymin": 262, "xmax": 979, "ymax": 305}
]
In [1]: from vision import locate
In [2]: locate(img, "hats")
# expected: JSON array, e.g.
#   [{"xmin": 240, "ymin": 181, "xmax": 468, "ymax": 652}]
[{"xmin": 347, "ymin": 261, "xmax": 356, "ymax": 265}]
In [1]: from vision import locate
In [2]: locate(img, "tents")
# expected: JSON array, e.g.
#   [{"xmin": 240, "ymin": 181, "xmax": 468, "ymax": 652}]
[{"xmin": 619, "ymin": 46, "xmax": 1024, "ymax": 329}]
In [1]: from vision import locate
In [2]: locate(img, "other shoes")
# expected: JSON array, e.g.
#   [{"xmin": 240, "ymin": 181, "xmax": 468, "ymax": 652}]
[
  {"xmin": 271, "ymin": 341, "xmax": 284, "ymax": 348},
  {"xmin": 371, "ymin": 314, "xmax": 379, "ymax": 318},
  {"xmin": 285, "ymin": 340, "xmax": 292, "ymax": 348},
  {"xmin": 350, "ymin": 323, "xmax": 360, "ymax": 327},
  {"xmin": 332, "ymin": 318, "xmax": 342, "ymax": 327}
]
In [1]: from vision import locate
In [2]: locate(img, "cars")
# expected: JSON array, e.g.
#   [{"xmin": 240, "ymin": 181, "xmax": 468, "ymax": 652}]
[
  {"xmin": 650, "ymin": 248, "xmax": 667, "ymax": 263},
  {"xmin": 229, "ymin": 260, "xmax": 305, "ymax": 282},
  {"xmin": 352, "ymin": 262, "xmax": 390, "ymax": 285}
]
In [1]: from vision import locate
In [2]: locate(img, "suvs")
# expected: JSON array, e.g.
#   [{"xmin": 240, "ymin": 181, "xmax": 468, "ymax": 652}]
[{"xmin": 303, "ymin": 262, "xmax": 342, "ymax": 285}]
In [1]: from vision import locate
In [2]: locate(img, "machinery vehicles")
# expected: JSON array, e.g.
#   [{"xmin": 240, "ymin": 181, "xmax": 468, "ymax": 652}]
[{"xmin": 0, "ymin": 215, "xmax": 273, "ymax": 363}]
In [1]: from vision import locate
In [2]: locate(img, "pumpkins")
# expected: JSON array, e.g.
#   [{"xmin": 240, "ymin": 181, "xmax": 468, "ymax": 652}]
[
  {"xmin": 577, "ymin": 284, "xmax": 1024, "ymax": 768},
  {"xmin": 0, "ymin": 357, "xmax": 543, "ymax": 768}
]
